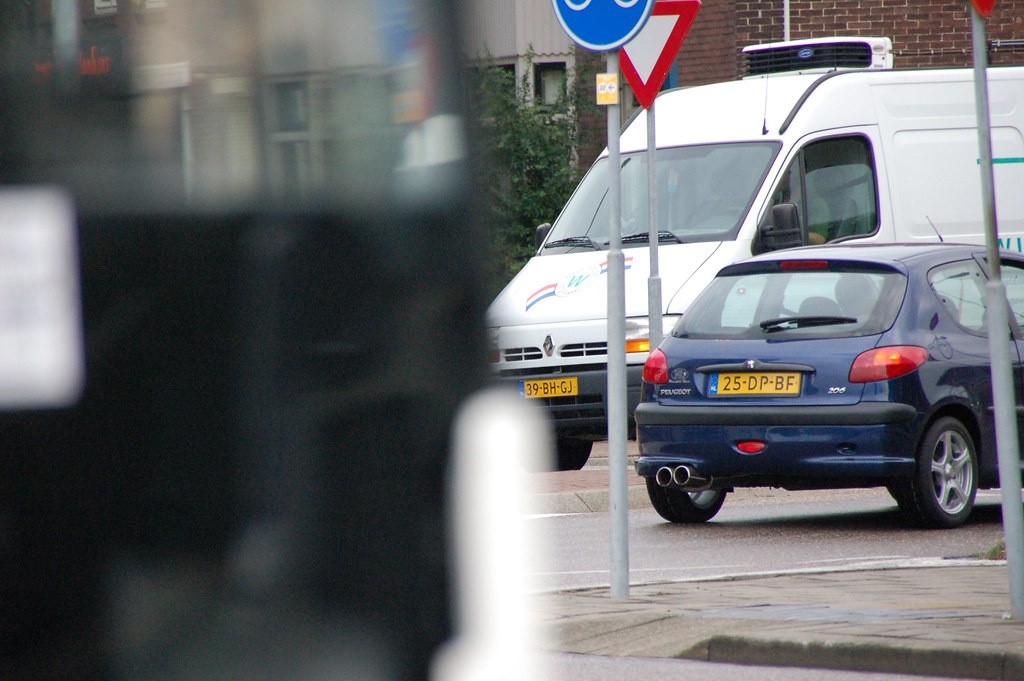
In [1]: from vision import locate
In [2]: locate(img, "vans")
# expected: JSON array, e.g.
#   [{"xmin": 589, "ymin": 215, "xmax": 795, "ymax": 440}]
[{"xmin": 484, "ymin": 36, "xmax": 1024, "ymax": 472}]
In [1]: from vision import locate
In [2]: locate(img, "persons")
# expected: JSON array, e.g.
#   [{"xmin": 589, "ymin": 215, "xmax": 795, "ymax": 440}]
[{"xmin": 771, "ymin": 156, "xmax": 830, "ymax": 244}]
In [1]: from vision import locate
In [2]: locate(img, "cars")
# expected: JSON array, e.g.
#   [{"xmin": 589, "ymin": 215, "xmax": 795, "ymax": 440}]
[{"xmin": 631, "ymin": 237, "xmax": 1023, "ymax": 529}]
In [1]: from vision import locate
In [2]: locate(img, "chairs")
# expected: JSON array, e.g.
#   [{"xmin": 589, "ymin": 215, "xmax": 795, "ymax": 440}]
[
  {"xmin": 798, "ymin": 296, "xmax": 840, "ymax": 326},
  {"xmin": 814, "ymin": 164, "xmax": 856, "ymax": 241}
]
